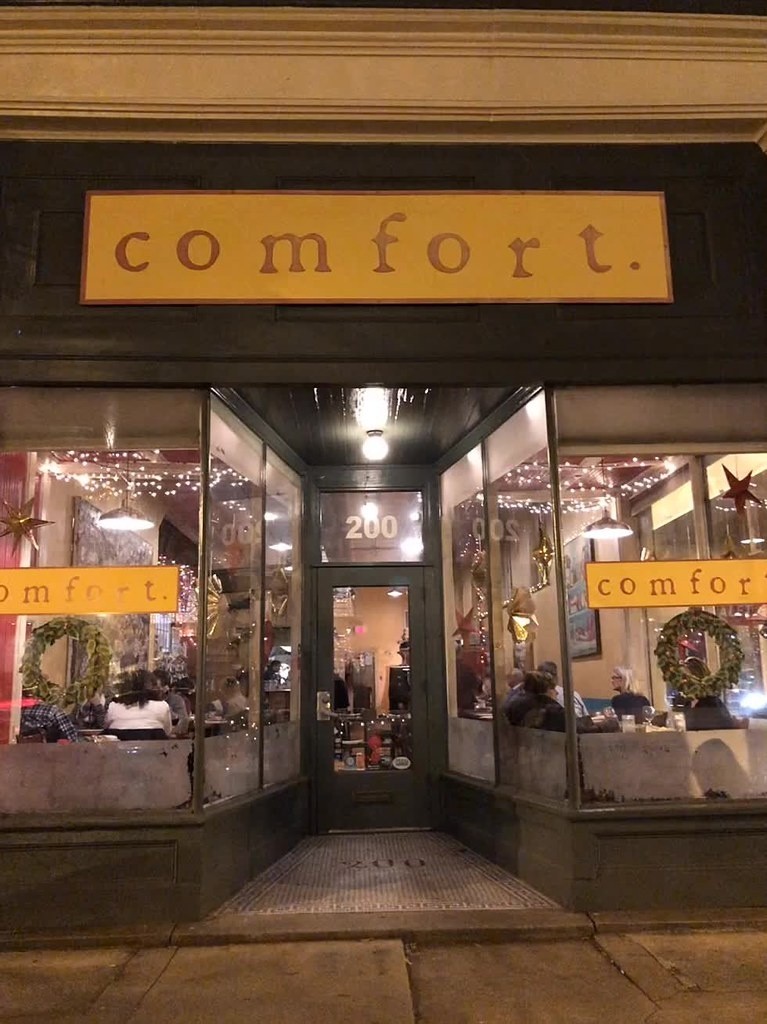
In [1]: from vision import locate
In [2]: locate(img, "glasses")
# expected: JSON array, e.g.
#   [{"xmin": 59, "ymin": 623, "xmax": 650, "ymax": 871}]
[{"xmin": 612, "ymin": 676, "xmax": 621, "ymax": 679}]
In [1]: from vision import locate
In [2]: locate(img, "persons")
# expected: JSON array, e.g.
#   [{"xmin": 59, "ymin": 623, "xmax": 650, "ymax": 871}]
[
  {"xmin": 335, "ymin": 671, "xmax": 350, "ymax": 735},
  {"xmin": 18, "ymin": 669, "xmax": 271, "ymax": 743},
  {"xmin": 454, "ymin": 644, "xmax": 482, "ymax": 717},
  {"xmin": 610, "ymin": 666, "xmax": 650, "ymax": 724},
  {"xmin": 652, "ymin": 657, "xmax": 734, "ymax": 731},
  {"xmin": 502, "ymin": 661, "xmax": 620, "ymax": 733},
  {"xmin": 263, "ymin": 659, "xmax": 281, "ymax": 686}
]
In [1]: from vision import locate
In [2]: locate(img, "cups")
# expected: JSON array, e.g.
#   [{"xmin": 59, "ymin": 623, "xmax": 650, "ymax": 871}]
[
  {"xmin": 604, "ymin": 707, "xmax": 615, "ymax": 718},
  {"xmin": 622, "ymin": 715, "xmax": 635, "ymax": 733}
]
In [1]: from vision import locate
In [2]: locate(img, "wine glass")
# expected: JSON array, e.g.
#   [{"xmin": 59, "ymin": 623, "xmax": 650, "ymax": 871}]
[{"xmin": 642, "ymin": 706, "xmax": 655, "ymax": 726}]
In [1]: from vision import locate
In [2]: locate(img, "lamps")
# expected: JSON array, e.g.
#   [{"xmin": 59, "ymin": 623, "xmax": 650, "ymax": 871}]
[
  {"xmin": 96, "ymin": 452, "xmax": 155, "ymax": 531},
  {"xmin": 582, "ymin": 459, "xmax": 635, "ymax": 539}
]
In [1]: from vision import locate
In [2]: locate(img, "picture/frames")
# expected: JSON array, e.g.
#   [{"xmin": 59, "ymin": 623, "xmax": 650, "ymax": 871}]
[{"xmin": 560, "ymin": 534, "xmax": 601, "ymax": 659}]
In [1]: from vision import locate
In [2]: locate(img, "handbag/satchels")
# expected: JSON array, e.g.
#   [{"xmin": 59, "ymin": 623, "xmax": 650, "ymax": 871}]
[{"xmin": 574, "ymin": 696, "xmax": 600, "ymax": 734}]
